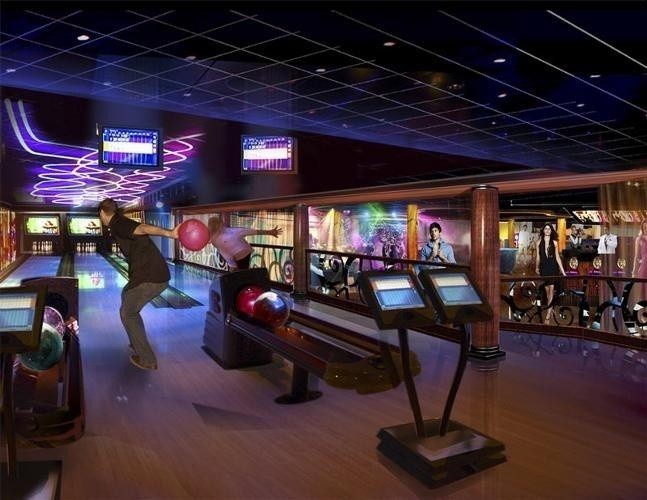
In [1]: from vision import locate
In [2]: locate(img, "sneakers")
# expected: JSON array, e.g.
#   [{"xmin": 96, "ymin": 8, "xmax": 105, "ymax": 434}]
[{"xmin": 126, "ymin": 343, "xmax": 158, "ymax": 370}]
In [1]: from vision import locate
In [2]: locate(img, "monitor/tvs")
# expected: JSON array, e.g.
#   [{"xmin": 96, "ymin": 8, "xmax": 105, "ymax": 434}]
[
  {"xmin": 0, "ymin": 285, "xmax": 47, "ymax": 354},
  {"xmin": 418, "ymin": 267, "xmax": 494, "ymax": 324},
  {"xmin": 98, "ymin": 121, "xmax": 164, "ymax": 171},
  {"xmin": 67, "ymin": 215, "xmax": 104, "ymax": 237},
  {"xmin": 24, "ymin": 214, "xmax": 60, "ymax": 236},
  {"xmin": 240, "ymin": 134, "xmax": 298, "ymax": 176},
  {"xmin": 359, "ymin": 269, "xmax": 437, "ymax": 330}
]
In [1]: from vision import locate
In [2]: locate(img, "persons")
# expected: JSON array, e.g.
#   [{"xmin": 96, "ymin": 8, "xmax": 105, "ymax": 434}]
[
  {"xmin": 517, "ymin": 224, "xmax": 531, "ymax": 264},
  {"xmin": 536, "ymin": 222, "xmax": 565, "ymax": 325},
  {"xmin": 627, "ymin": 218, "xmax": 647, "ymax": 314},
  {"xmin": 209, "ymin": 217, "xmax": 284, "ymax": 270},
  {"xmin": 420, "ymin": 222, "xmax": 457, "ymax": 271},
  {"xmin": 570, "ymin": 225, "xmax": 618, "ymax": 255},
  {"xmin": 99, "ymin": 200, "xmax": 181, "ymax": 372}
]
